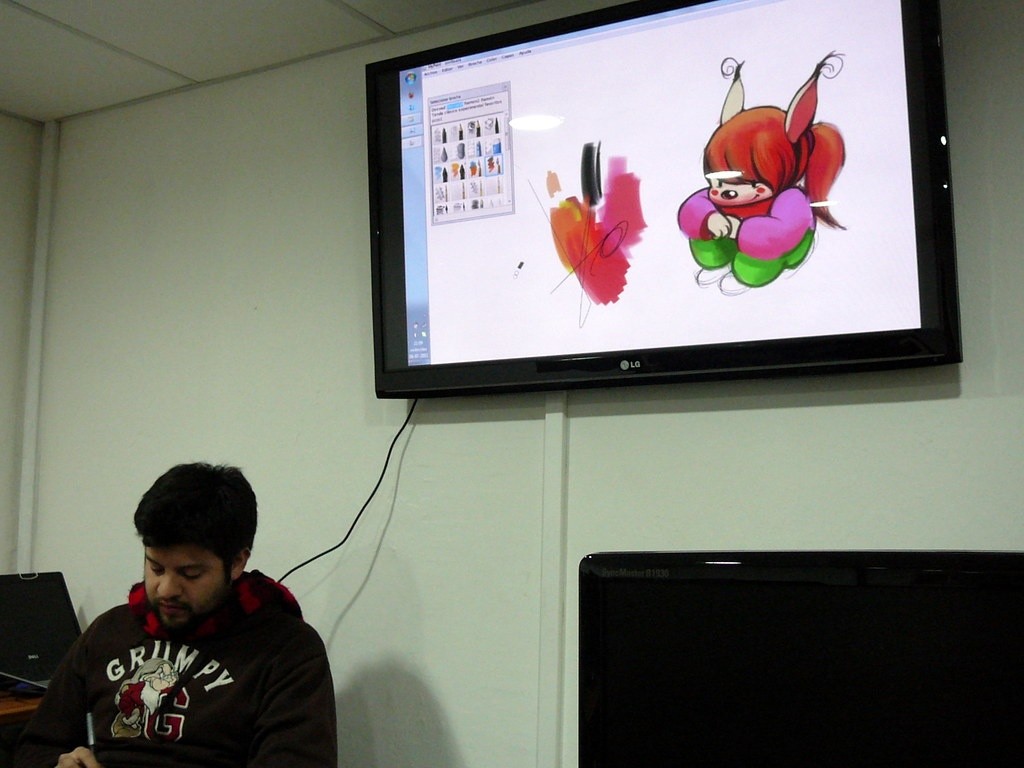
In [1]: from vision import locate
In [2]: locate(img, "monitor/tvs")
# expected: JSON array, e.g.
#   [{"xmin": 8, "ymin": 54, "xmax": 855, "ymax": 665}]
[
  {"xmin": 367, "ymin": 0, "xmax": 962, "ymax": 401},
  {"xmin": 577, "ymin": 550, "xmax": 1024, "ymax": 767}
]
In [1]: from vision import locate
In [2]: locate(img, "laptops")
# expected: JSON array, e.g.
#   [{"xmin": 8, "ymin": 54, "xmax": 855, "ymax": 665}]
[{"xmin": 0, "ymin": 572, "xmax": 82, "ymax": 690}]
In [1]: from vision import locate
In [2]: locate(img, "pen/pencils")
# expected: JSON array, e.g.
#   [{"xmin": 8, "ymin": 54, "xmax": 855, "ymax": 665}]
[{"xmin": 84, "ymin": 696, "xmax": 96, "ymax": 760}]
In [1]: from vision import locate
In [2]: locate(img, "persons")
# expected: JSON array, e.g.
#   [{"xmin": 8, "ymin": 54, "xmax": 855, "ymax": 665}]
[{"xmin": 13, "ymin": 462, "xmax": 340, "ymax": 768}]
